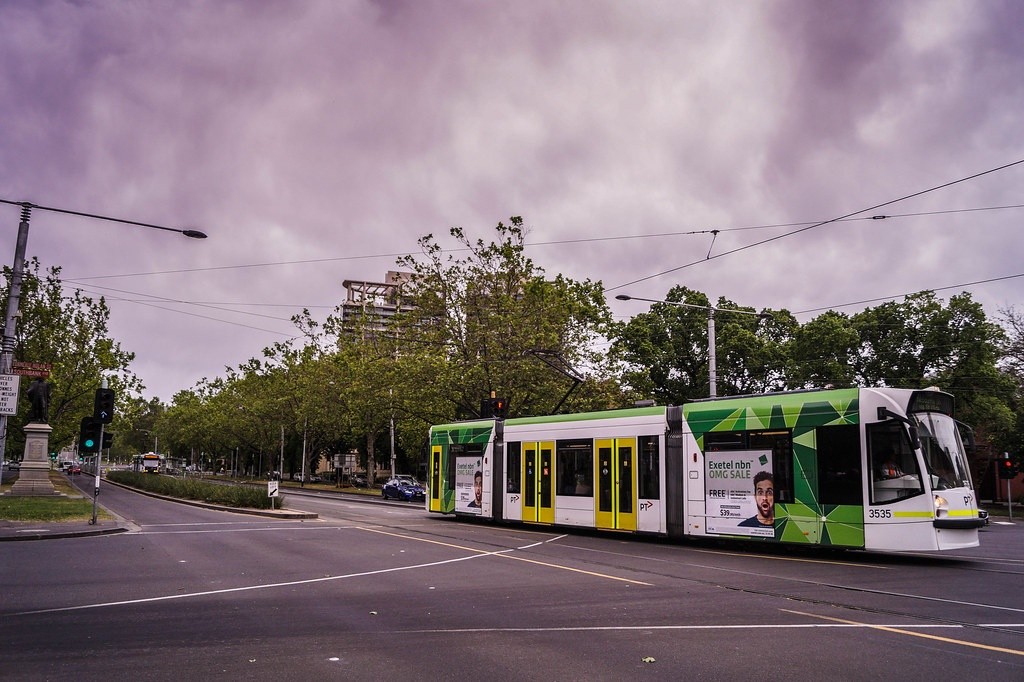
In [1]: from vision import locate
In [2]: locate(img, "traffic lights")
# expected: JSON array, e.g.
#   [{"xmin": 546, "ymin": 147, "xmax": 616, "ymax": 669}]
[
  {"xmin": 103, "ymin": 432, "xmax": 114, "ymax": 449},
  {"xmin": 1004, "ymin": 459, "xmax": 1014, "ymax": 479},
  {"xmin": 51, "ymin": 452, "xmax": 56, "ymax": 460},
  {"xmin": 491, "ymin": 401, "xmax": 498, "ymax": 416},
  {"xmin": 79, "ymin": 417, "xmax": 101, "ymax": 452},
  {"xmin": 80, "ymin": 458, "xmax": 83, "ymax": 461},
  {"xmin": 498, "ymin": 399, "xmax": 506, "ymax": 416},
  {"xmin": 94, "ymin": 388, "xmax": 115, "ymax": 424}
]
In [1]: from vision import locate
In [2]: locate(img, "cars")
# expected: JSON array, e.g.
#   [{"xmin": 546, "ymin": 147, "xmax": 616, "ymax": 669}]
[
  {"xmin": 305, "ymin": 474, "xmax": 322, "ymax": 483},
  {"xmin": 293, "ymin": 473, "xmax": 306, "ymax": 481},
  {"xmin": 62, "ymin": 461, "xmax": 73, "ymax": 472},
  {"xmin": 381, "ymin": 478, "xmax": 426, "ymax": 502},
  {"xmin": 978, "ymin": 506, "xmax": 989, "ymax": 525},
  {"xmin": 388, "ymin": 475, "xmax": 420, "ymax": 486},
  {"xmin": 348, "ymin": 474, "xmax": 367, "ymax": 484},
  {"xmin": 68, "ymin": 465, "xmax": 80, "ymax": 475}
]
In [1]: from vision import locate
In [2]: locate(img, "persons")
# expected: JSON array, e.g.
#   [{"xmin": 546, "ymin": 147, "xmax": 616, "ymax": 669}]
[
  {"xmin": 737, "ymin": 472, "xmax": 774, "ymax": 529},
  {"xmin": 880, "ymin": 448, "xmax": 910, "ymax": 498},
  {"xmin": 468, "ymin": 471, "xmax": 482, "ymax": 508}
]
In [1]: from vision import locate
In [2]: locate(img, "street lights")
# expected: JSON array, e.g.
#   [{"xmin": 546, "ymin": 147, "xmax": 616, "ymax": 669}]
[
  {"xmin": 329, "ymin": 382, "xmax": 395, "ymax": 480},
  {"xmin": 615, "ymin": 295, "xmax": 773, "ymax": 397},
  {"xmin": 144, "ymin": 431, "xmax": 157, "ymax": 454},
  {"xmin": 0, "ymin": 199, "xmax": 207, "ymax": 484},
  {"xmin": 295, "ymin": 412, "xmax": 307, "ymax": 487},
  {"xmin": 238, "ymin": 407, "xmax": 284, "ymax": 482}
]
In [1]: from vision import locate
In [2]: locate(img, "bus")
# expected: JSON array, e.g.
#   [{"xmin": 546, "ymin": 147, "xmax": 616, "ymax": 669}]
[
  {"xmin": 425, "ymin": 386, "xmax": 986, "ymax": 552},
  {"xmin": 132, "ymin": 452, "xmax": 162, "ymax": 475}
]
